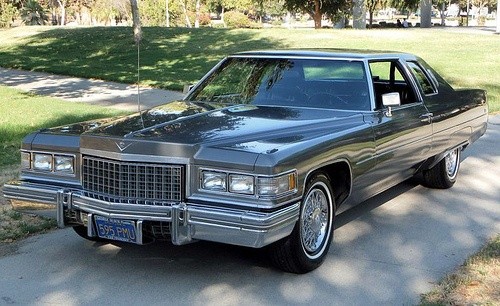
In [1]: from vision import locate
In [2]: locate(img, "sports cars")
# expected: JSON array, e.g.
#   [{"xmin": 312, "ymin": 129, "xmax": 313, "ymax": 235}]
[{"xmin": 2, "ymin": 47, "xmax": 489, "ymax": 273}]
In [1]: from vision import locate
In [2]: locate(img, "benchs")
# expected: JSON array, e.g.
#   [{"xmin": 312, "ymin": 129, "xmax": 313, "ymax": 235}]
[{"xmin": 303, "ymin": 80, "xmax": 407, "ymax": 109}]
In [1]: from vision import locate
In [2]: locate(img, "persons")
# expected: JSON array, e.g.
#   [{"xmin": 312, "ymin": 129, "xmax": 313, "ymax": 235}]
[
  {"xmin": 402, "ymin": 18, "xmax": 409, "ymax": 28},
  {"xmin": 396, "ymin": 19, "xmax": 402, "ymax": 27}
]
